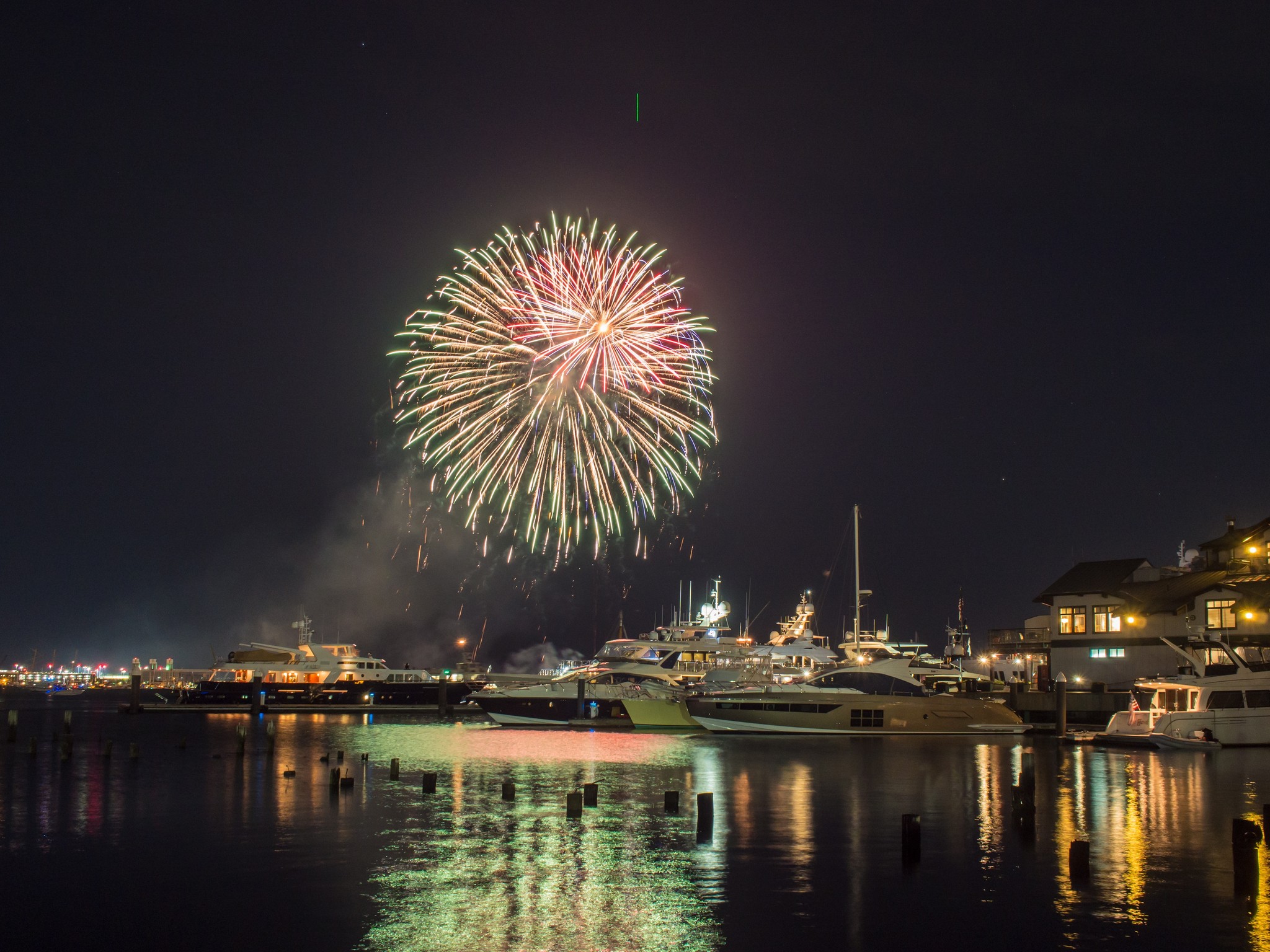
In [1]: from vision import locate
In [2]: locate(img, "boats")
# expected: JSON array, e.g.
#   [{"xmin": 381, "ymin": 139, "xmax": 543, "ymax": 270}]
[
  {"xmin": 0, "ymin": 494, "xmax": 1037, "ymax": 739},
  {"xmin": 1066, "ymin": 638, "xmax": 1270, "ymax": 754}
]
